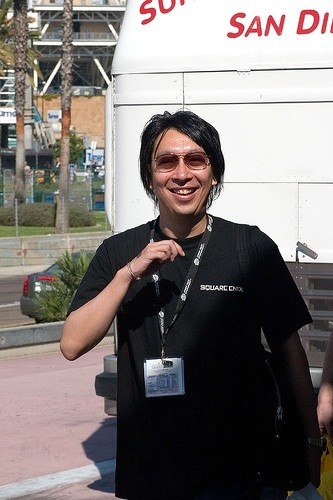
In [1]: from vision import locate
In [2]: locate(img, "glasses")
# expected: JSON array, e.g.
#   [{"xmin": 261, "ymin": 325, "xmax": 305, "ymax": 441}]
[{"xmin": 150, "ymin": 151, "xmax": 211, "ymax": 173}]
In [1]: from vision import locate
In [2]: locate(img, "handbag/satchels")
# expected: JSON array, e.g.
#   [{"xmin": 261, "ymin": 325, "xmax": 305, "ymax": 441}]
[
  {"xmin": 315, "ymin": 438, "xmax": 332, "ymax": 500},
  {"xmin": 262, "ymin": 354, "xmax": 309, "ymax": 491}
]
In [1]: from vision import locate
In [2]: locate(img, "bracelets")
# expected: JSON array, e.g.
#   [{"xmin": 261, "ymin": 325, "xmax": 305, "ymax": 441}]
[{"xmin": 126, "ymin": 262, "xmax": 141, "ymax": 280}]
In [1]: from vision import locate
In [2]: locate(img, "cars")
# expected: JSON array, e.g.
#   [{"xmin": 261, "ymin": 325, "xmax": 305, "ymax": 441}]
[{"xmin": 21, "ymin": 250, "xmax": 102, "ymax": 324}]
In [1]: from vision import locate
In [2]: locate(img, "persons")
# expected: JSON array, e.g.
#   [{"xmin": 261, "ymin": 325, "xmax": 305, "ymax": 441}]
[
  {"xmin": 59, "ymin": 111, "xmax": 322, "ymax": 500},
  {"xmin": 317, "ymin": 331, "xmax": 333, "ymax": 445}
]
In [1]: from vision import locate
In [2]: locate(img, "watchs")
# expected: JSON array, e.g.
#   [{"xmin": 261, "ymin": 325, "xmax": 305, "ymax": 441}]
[{"xmin": 305, "ymin": 435, "xmax": 327, "ymax": 452}]
